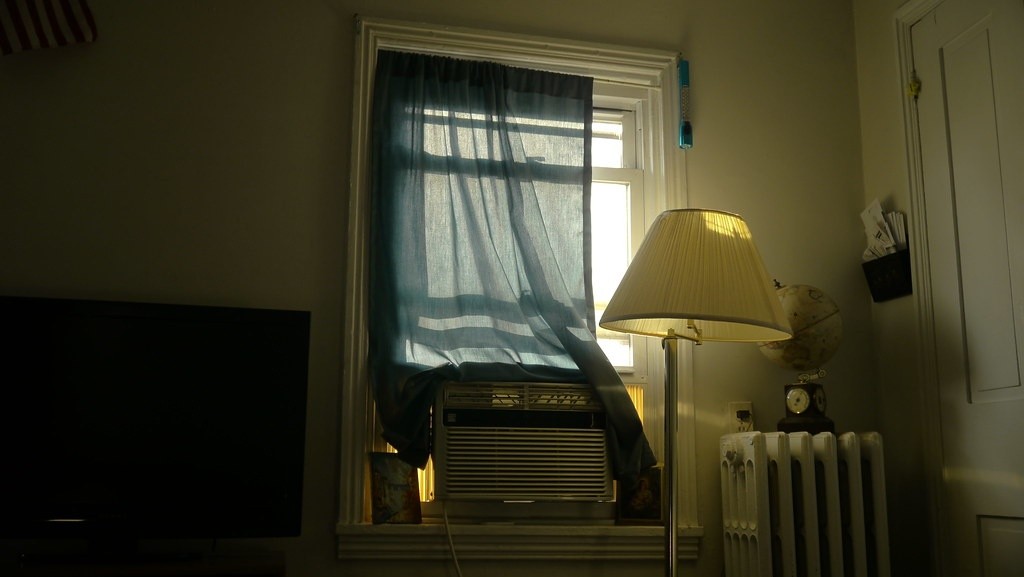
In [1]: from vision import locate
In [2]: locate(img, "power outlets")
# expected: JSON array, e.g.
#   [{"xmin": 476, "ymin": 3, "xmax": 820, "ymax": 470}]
[{"xmin": 728, "ymin": 401, "xmax": 754, "ymax": 433}]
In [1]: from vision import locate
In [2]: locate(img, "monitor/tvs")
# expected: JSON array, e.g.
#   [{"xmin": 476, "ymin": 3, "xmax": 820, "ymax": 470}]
[{"xmin": 0, "ymin": 294, "xmax": 311, "ymax": 565}]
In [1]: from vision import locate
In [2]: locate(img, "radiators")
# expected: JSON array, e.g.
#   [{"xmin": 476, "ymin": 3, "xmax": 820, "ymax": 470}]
[{"xmin": 721, "ymin": 431, "xmax": 891, "ymax": 577}]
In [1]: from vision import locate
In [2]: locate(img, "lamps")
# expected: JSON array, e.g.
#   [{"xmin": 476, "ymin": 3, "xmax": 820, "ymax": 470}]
[{"xmin": 600, "ymin": 206, "xmax": 795, "ymax": 577}]
